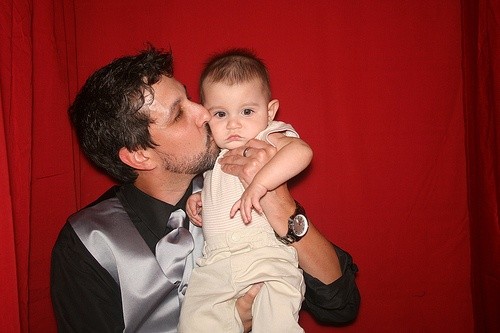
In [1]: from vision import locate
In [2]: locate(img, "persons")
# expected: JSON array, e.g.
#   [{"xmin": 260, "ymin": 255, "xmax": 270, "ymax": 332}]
[{"xmin": 48, "ymin": 42, "xmax": 361, "ymax": 333}]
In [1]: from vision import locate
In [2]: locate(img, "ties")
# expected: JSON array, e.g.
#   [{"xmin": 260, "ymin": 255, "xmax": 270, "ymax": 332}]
[{"xmin": 155, "ymin": 209, "xmax": 195, "ymax": 284}]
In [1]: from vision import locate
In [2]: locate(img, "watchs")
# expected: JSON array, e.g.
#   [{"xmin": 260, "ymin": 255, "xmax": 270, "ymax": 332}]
[{"xmin": 274, "ymin": 200, "xmax": 308, "ymax": 246}]
